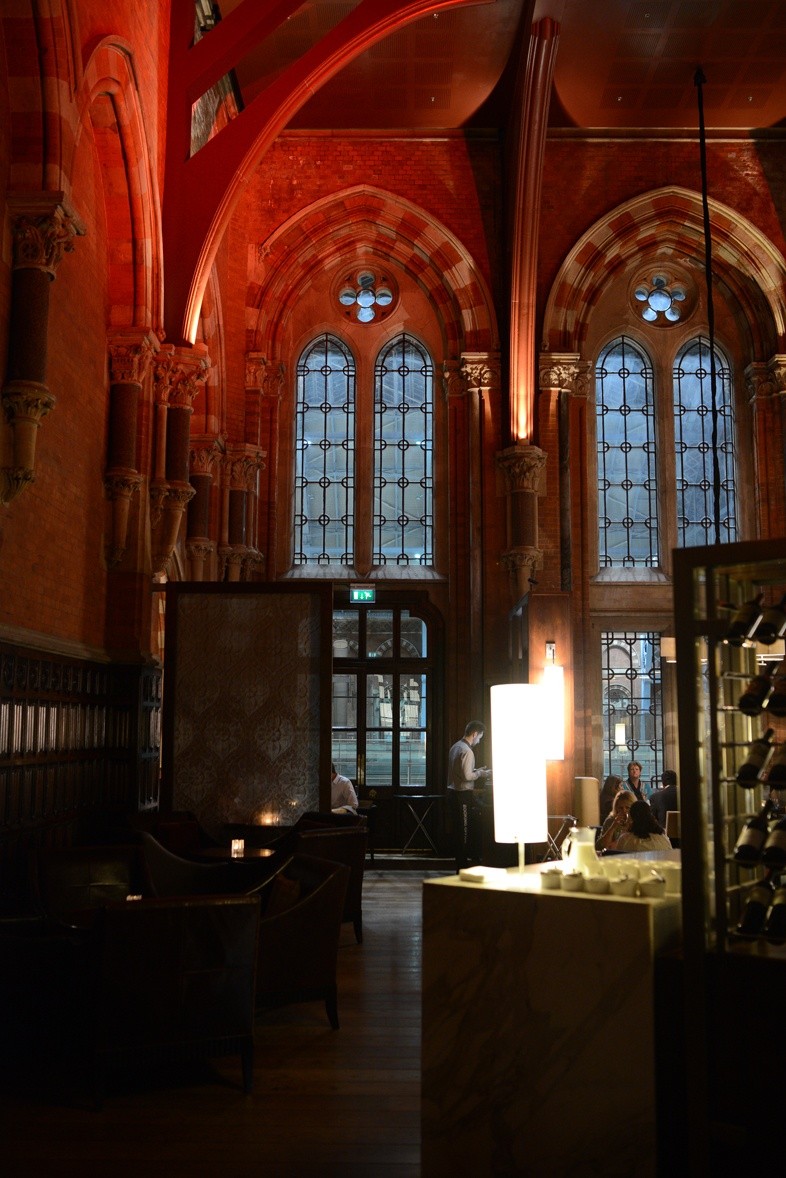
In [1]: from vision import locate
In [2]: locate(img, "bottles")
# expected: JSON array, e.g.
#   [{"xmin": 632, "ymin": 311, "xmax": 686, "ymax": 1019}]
[
  {"xmin": 726, "ymin": 592, "xmax": 765, "ymax": 648},
  {"xmin": 768, "ymin": 674, "xmax": 786, "ymax": 716},
  {"xmin": 733, "ymin": 799, "xmax": 774, "ymax": 868},
  {"xmin": 763, "ymin": 883, "xmax": 786, "ymax": 945},
  {"xmin": 739, "ymin": 661, "xmax": 779, "ymax": 716},
  {"xmin": 762, "ymin": 817, "xmax": 786, "ymax": 870},
  {"xmin": 736, "ymin": 868, "xmax": 776, "ymax": 943},
  {"xmin": 757, "ymin": 594, "xmax": 786, "ymax": 644},
  {"xmin": 736, "ymin": 728, "xmax": 774, "ymax": 789},
  {"xmin": 767, "ymin": 740, "xmax": 786, "ymax": 790}
]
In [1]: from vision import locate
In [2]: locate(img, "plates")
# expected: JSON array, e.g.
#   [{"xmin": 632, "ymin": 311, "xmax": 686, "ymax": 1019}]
[{"xmin": 473, "ymin": 769, "xmax": 491, "ymax": 773}]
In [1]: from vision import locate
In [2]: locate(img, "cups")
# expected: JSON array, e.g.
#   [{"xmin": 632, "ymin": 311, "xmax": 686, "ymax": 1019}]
[
  {"xmin": 583, "ymin": 877, "xmax": 608, "ymax": 893},
  {"xmin": 639, "ymin": 879, "xmax": 667, "ymax": 897},
  {"xmin": 661, "ymin": 861, "xmax": 682, "ymax": 893},
  {"xmin": 641, "ymin": 860, "xmax": 661, "ymax": 878},
  {"xmin": 608, "ymin": 878, "xmax": 638, "ymax": 897},
  {"xmin": 621, "ymin": 859, "xmax": 640, "ymax": 879},
  {"xmin": 603, "ymin": 860, "xmax": 620, "ymax": 875},
  {"xmin": 540, "ymin": 871, "xmax": 561, "ymax": 889},
  {"xmin": 559, "ymin": 875, "xmax": 583, "ymax": 890}
]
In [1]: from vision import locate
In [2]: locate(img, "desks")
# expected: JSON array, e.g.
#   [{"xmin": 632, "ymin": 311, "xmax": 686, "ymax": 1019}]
[
  {"xmin": 543, "ymin": 813, "xmax": 576, "ymax": 862},
  {"xmin": 418, "ymin": 847, "xmax": 681, "ymax": 1178},
  {"xmin": 392, "ymin": 793, "xmax": 446, "ymax": 856},
  {"xmin": 194, "ymin": 845, "xmax": 277, "ymax": 893}
]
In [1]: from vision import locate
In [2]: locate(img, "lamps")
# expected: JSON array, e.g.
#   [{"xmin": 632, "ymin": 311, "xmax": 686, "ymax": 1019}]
[
  {"xmin": 486, "ymin": 682, "xmax": 553, "ymax": 870},
  {"xmin": 540, "ymin": 641, "xmax": 565, "ymax": 764}
]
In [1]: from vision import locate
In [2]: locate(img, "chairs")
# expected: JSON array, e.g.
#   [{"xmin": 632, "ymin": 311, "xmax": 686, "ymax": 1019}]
[{"xmin": 0, "ymin": 811, "xmax": 371, "ymax": 1111}]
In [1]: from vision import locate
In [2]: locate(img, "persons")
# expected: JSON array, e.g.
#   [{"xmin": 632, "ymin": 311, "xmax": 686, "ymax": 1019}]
[
  {"xmin": 447, "ymin": 719, "xmax": 492, "ymax": 843},
  {"xmin": 600, "ymin": 761, "xmax": 677, "ymax": 851},
  {"xmin": 331, "ymin": 764, "xmax": 358, "ymax": 813}
]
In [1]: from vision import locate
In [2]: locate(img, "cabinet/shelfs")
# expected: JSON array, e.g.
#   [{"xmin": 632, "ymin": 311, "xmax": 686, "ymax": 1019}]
[{"xmin": 672, "ymin": 538, "xmax": 786, "ymax": 1178}]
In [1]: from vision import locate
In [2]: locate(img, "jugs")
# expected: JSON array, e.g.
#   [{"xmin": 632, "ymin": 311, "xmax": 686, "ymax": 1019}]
[{"xmin": 560, "ymin": 826, "xmax": 605, "ymax": 877}]
